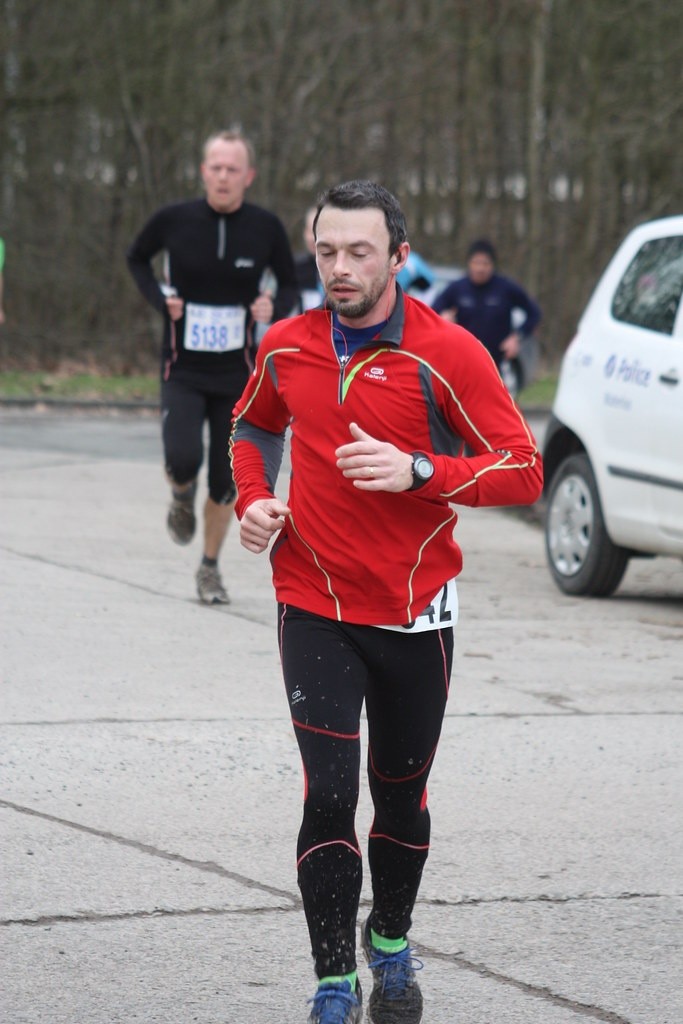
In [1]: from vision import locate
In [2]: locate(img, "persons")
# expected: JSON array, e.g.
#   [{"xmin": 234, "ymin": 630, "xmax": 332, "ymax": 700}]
[
  {"xmin": 291, "ymin": 203, "xmax": 433, "ymax": 318},
  {"xmin": 128, "ymin": 127, "xmax": 298, "ymax": 605},
  {"xmin": 428, "ymin": 238, "xmax": 539, "ymax": 381},
  {"xmin": 227, "ymin": 182, "xmax": 543, "ymax": 1024}
]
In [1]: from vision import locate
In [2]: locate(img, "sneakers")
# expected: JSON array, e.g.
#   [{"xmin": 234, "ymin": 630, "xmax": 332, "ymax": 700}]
[
  {"xmin": 360, "ymin": 913, "xmax": 423, "ymax": 1024},
  {"xmin": 167, "ymin": 478, "xmax": 196, "ymax": 545},
  {"xmin": 196, "ymin": 564, "xmax": 230, "ymax": 605},
  {"xmin": 306, "ymin": 970, "xmax": 363, "ymax": 1024}
]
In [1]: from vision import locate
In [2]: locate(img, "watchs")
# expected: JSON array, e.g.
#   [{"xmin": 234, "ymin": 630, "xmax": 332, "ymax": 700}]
[{"xmin": 406, "ymin": 452, "xmax": 434, "ymax": 491}]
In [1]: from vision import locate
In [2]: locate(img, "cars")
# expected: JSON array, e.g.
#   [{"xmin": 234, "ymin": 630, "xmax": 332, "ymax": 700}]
[{"xmin": 538, "ymin": 218, "xmax": 683, "ymax": 603}]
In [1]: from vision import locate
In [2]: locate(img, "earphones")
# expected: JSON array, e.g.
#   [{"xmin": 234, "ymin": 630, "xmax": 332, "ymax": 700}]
[{"xmin": 394, "ymin": 252, "xmax": 401, "ymax": 266}]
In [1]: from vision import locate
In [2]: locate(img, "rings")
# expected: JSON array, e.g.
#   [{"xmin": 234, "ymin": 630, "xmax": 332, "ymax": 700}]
[{"xmin": 370, "ymin": 466, "xmax": 373, "ymax": 478}]
